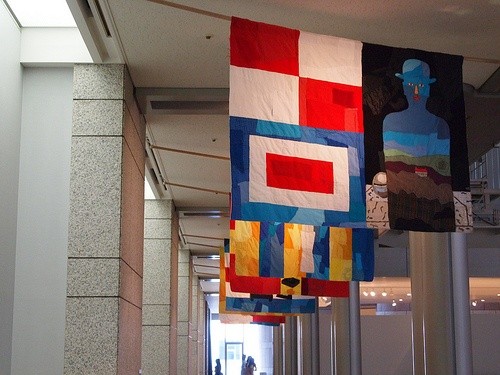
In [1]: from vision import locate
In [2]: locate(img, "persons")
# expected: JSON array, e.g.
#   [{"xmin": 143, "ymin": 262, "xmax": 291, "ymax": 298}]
[
  {"xmin": 241, "ymin": 354, "xmax": 257, "ymax": 375},
  {"xmin": 214, "ymin": 358, "xmax": 223, "ymax": 375}
]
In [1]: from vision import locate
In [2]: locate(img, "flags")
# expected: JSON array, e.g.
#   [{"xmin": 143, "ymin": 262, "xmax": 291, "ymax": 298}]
[
  {"xmin": 219, "ymin": 255, "xmax": 318, "ymax": 329},
  {"xmin": 223, "ymin": 236, "xmax": 350, "ymax": 299},
  {"xmin": 229, "ymin": 14, "xmax": 474, "ymax": 233},
  {"xmin": 227, "ymin": 190, "xmax": 376, "ymax": 282}
]
